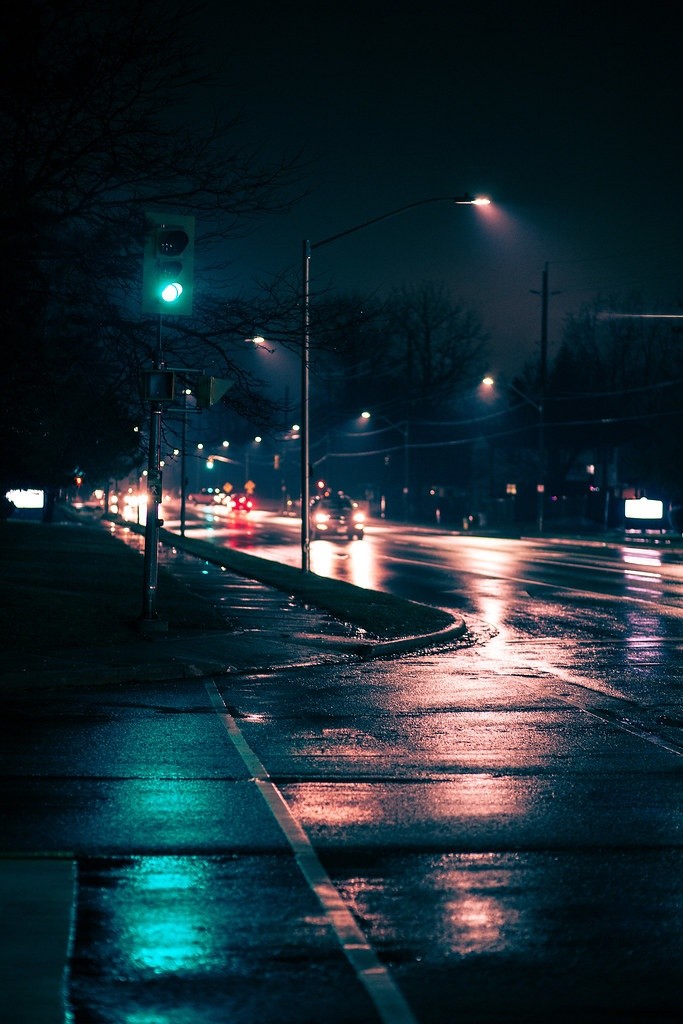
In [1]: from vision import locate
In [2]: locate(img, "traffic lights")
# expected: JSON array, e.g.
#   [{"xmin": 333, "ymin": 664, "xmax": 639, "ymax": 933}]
[
  {"xmin": 198, "ymin": 376, "xmax": 236, "ymax": 409},
  {"xmin": 141, "ymin": 212, "xmax": 195, "ymax": 318},
  {"xmin": 143, "ymin": 369, "xmax": 175, "ymax": 402}
]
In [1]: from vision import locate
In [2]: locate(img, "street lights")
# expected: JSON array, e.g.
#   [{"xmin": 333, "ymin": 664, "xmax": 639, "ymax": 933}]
[
  {"xmin": 359, "ymin": 411, "xmax": 410, "ymax": 523},
  {"xmin": 301, "ymin": 192, "xmax": 490, "ymax": 574},
  {"xmin": 481, "ymin": 376, "xmax": 545, "ymax": 532}
]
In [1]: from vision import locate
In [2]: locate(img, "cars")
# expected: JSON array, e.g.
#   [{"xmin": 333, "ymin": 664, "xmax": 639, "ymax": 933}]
[
  {"xmin": 308, "ymin": 491, "xmax": 364, "ymax": 540},
  {"xmin": 227, "ymin": 493, "xmax": 256, "ymax": 514}
]
